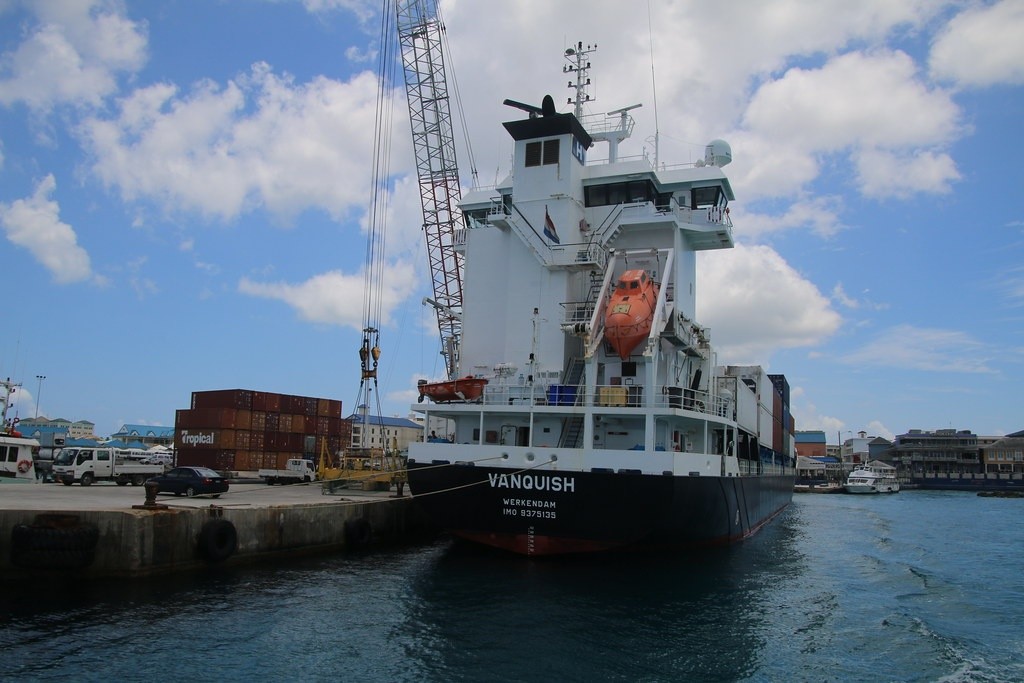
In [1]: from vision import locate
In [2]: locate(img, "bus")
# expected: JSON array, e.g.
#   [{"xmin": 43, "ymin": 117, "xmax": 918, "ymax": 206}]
[{"xmin": 114, "ymin": 444, "xmax": 174, "ymax": 465}]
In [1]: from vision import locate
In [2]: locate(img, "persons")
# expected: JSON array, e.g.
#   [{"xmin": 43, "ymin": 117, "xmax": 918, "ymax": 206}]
[{"xmin": 432, "ymin": 431, "xmax": 437, "ymax": 438}]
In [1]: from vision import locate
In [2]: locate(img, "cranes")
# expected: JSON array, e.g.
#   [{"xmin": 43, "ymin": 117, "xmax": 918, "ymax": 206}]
[{"xmin": 316, "ymin": 1, "xmax": 472, "ymax": 493}]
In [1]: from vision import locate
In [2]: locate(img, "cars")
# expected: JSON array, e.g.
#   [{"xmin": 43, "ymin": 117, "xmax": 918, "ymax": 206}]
[{"xmin": 145, "ymin": 466, "xmax": 229, "ymax": 499}]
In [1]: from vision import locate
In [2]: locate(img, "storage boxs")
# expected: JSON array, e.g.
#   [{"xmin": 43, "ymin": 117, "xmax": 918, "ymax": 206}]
[
  {"xmin": 171, "ymin": 388, "xmax": 354, "ymax": 472},
  {"xmin": 711, "ymin": 364, "xmax": 796, "ymax": 465}
]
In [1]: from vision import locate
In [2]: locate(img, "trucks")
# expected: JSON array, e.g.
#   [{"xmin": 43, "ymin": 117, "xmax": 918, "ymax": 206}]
[
  {"xmin": 52, "ymin": 446, "xmax": 165, "ymax": 487},
  {"xmin": 259, "ymin": 459, "xmax": 317, "ymax": 486}
]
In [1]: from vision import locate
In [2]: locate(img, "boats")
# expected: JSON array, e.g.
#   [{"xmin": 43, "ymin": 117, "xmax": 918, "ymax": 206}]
[
  {"xmin": 358, "ymin": 0, "xmax": 797, "ymax": 567},
  {"xmin": 0, "ymin": 377, "xmax": 44, "ymax": 484},
  {"xmin": 843, "ymin": 454, "xmax": 900, "ymax": 494},
  {"xmin": 793, "ymin": 456, "xmax": 841, "ymax": 493}
]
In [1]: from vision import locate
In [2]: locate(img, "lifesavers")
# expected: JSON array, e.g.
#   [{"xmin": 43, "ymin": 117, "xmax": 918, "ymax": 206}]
[{"xmin": 18, "ymin": 460, "xmax": 30, "ymax": 474}]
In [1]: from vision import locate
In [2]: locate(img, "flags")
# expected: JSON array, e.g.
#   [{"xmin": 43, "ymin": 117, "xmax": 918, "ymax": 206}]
[{"xmin": 543, "ymin": 211, "xmax": 560, "ymax": 244}]
